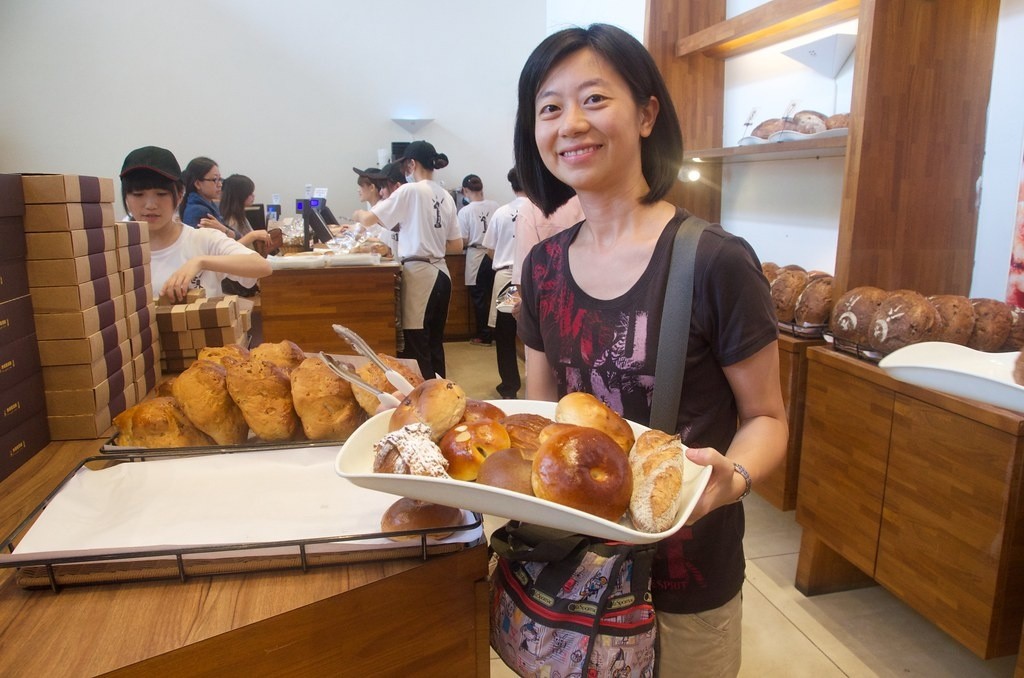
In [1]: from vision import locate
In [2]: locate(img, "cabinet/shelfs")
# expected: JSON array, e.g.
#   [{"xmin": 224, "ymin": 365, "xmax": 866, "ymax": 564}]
[
  {"xmin": 793, "ymin": 343, "xmax": 1023, "ymax": 666},
  {"xmin": 642, "ymin": 1, "xmax": 1003, "ymax": 510}
]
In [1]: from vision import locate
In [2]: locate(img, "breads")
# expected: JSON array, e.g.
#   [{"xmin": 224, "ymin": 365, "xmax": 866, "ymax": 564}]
[
  {"xmin": 750, "ymin": 111, "xmax": 850, "ymax": 139},
  {"xmin": 757, "ymin": 261, "xmax": 1024, "ymax": 389},
  {"xmin": 372, "ymin": 378, "xmax": 684, "ymax": 541},
  {"xmin": 275, "ymin": 223, "xmax": 396, "ymax": 259},
  {"xmin": 110, "ymin": 338, "xmax": 426, "ymax": 451}
]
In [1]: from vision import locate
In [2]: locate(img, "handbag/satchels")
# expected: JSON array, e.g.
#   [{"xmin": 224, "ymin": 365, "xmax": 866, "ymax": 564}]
[{"xmin": 486, "ymin": 524, "xmax": 657, "ymax": 678}]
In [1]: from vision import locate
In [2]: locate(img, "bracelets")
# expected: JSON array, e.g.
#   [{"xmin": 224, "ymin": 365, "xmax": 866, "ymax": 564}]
[
  {"xmin": 225, "ymin": 228, "xmax": 229, "ymax": 234},
  {"xmin": 733, "ymin": 462, "xmax": 753, "ymax": 500}
]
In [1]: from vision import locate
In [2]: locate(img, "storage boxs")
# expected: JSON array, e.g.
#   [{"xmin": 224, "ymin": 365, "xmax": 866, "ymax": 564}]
[{"xmin": 0, "ymin": 172, "xmax": 250, "ymax": 481}]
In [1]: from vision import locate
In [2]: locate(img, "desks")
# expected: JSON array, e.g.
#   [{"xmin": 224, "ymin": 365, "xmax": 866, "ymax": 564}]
[{"xmin": 0, "ymin": 359, "xmax": 492, "ymax": 678}]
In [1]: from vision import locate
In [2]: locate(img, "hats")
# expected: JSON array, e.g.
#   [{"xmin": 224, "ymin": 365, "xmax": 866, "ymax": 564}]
[
  {"xmin": 460, "ymin": 174, "xmax": 481, "ymax": 193},
  {"xmin": 353, "ymin": 167, "xmax": 383, "ymax": 188},
  {"xmin": 393, "ymin": 141, "xmax": 436, "ymax": 164},
  {"xmin": 120, "ymin": 146, "xmax": 181, "ymax": 181},
  {"xmin": 368, "ymin": 163, "xmax": 402, "ymax": 179}
]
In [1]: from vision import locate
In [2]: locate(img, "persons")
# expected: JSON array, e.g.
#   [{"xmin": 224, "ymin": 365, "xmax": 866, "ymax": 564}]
[
  {"xmin": 182, "ymin": 156, "xmax": 240, "ymax": 298},
  {"xmin": 510, "ymin": 191, "xmax": 586, "ymax": 304},
  {"xmin": 120, "ymin": 146, "xmax": 273, "ymax": 300},
  {"xmin": 481, "ymin": 166, "xmax": 525, "ymax": 400},
  {"xmin": 458, "ymin": 175, "xmax": 502, "ymax": 345},
  {"xmin": 513, "ymin": 22, "xmax": 790, "ymax": 678},
  {"xmin": 220, "ymin": 173, "xmax": 270, "ymax": 309},
  {"xmin": 351, "ymin": 140, "xmax": 464, "ymax": 384}
]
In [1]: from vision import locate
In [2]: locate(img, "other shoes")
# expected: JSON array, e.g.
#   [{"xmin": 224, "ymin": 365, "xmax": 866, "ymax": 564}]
[{"xmin": 469, "ymin": 338, "xmax": 492, "ymax": 346}]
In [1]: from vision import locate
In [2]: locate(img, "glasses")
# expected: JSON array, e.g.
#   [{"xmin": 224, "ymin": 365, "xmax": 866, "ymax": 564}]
[{"xmin": 199, "ymin": 177, "xmax": 221, "ymax": 184}]
